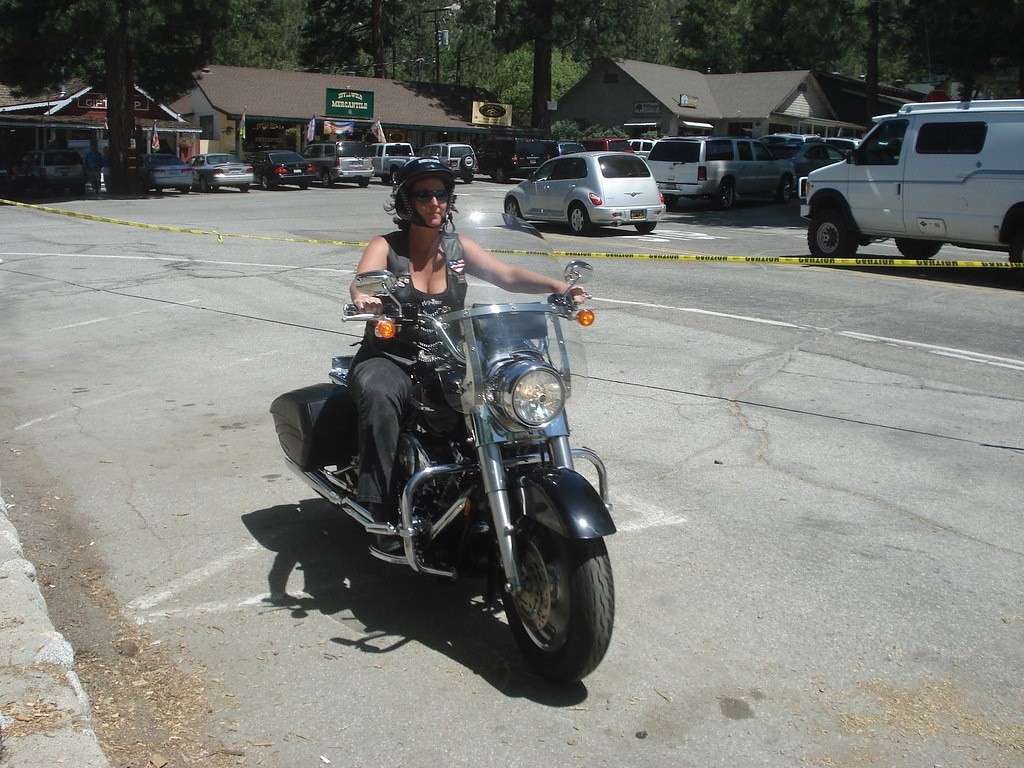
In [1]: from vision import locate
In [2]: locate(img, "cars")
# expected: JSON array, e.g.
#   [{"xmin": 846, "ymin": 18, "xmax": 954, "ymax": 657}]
[
  {"xmin": 8, "ymin": 147, "xmax": 88, "ymax": 196},
  {"xmin": 755, "ymin": 133, "xmax": 890, "ymax": 200},
  {"xmin": 183, "ymin": 152, "xmax": 255, "ymax": 193},
  {"xmin": 127, "ymin": 153, "xmax": 194, "ymax": 194},
  {"xmin": 243, "ymin": 149, "xmax": 317, "ymax": 190},
  {"xmin": 503, "ymin": 151, "xmax": 667, "ymax": 237}
]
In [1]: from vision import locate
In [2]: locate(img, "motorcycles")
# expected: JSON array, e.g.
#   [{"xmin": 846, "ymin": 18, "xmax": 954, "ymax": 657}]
[{"xmin": 266, "ymin": 209, "xmax": 624, "ymax": 686}]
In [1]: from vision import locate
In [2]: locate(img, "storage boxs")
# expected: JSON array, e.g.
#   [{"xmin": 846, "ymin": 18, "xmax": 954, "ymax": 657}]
[{"xmin": 269, "ymin": 382, "xmax": 355, "ymax": 472}]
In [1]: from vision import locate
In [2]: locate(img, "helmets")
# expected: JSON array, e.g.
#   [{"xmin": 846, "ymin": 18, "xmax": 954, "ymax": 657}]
[{"xmin": 390, "ymin": 156, "xmax": 455, "ymax": 217}]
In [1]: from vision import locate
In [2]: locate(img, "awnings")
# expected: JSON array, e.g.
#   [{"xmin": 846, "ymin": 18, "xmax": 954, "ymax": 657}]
[
  {"xmin": 679, "ymin": 120, "xmax": 713, "ymax": 130},
  {"xmin": 624, "ymin": 117, "xmax": 662, "ymax": 127}
]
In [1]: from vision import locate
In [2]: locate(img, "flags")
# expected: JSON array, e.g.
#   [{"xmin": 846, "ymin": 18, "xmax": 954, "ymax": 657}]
[
  {"xmin": 152, "ymin": 125, "xmax": 160, "ymax": 152},
  {"xmin": 307, "ymin": 117, "xmax": 315, "ymax": 141},
  {"xmin": 372, "ymin": 121, "xmax": 386, "ymax": 143},
  {"xmin": 240, "ymin": 112, "xmax": 245, "ymax": 139}
]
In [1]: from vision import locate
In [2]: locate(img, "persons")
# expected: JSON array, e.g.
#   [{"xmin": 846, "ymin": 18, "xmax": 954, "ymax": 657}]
[
  {"xmin": 85, "ymin": 144, "xmax": 112, "ymax": 192},
  {"xmin": 346, "ymin": 157, "xmax": 584, "ymax": 551}
]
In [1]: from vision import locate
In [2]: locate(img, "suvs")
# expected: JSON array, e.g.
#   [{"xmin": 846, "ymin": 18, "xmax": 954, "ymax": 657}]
[
  {"xmin": 645, "ymin": 134, "xmax": 797, "ymax": 212},
  {"xmin": 298, "ymin": 138, "xmax": 375, "ymax": 187},
  {"xmin": 366, "ymin": 141, "xmax": 422, "ymax": 186},
  {"xmin": 415, "ymin": 142, "xmax": 479, "ymax": 184},
  {"xmin": 796, "ymin": 97, "xmax": 1024, "ymax": 290},
  {"xmin": 529, "ymin": 134, "xmax": 662, "ymax": 167},
  {"xmin": 473, "ymin": 134, "xmax": 560, "ymax": 183}
]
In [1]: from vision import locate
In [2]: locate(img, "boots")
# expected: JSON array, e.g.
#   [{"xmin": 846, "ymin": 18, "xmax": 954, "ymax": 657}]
[{"xmin": 369, "ymin": 501, "xmax": 403, "ymax": 551}]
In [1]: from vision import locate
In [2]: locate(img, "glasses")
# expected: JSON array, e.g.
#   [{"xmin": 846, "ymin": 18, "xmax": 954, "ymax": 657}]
[{"xmin": 412, "ymin": 189, "xmax": 450, "ymax": 202}]
